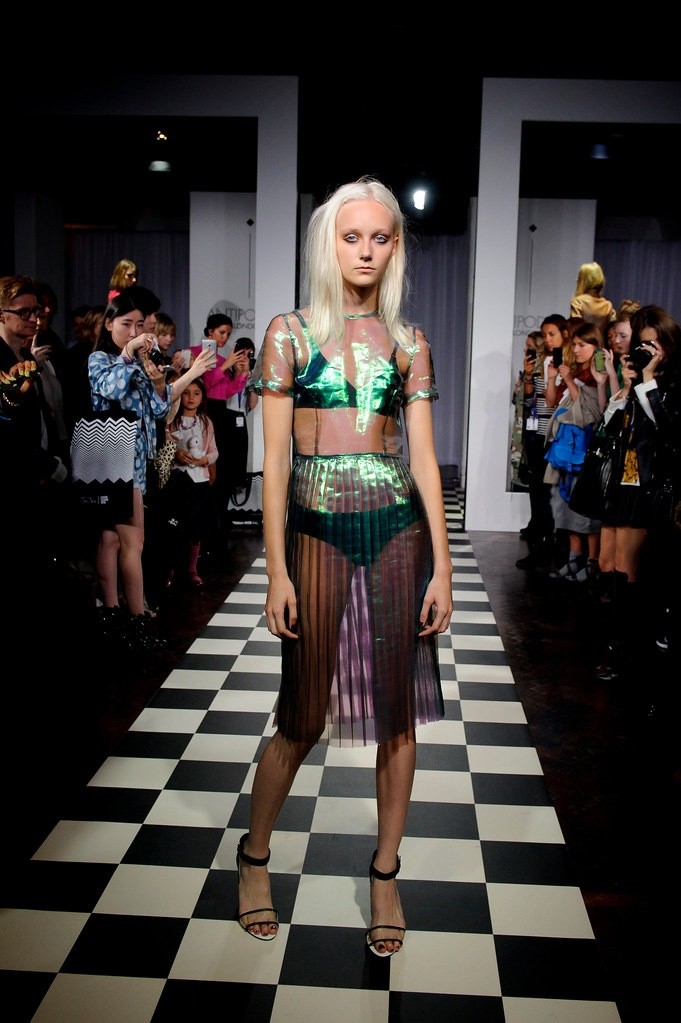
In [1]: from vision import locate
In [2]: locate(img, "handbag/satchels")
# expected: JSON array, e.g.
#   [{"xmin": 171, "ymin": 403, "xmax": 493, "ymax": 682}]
[{"xmin": 69, "ymin": 410, "xmax": 137, "ymax": 523}]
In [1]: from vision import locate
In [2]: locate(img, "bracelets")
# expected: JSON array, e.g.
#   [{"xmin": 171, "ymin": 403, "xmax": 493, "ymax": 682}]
[
  {"xmin": 525, "ymin": 378, "xmax": 533, "ymax": 385},
  {"xmin": 618, "ymin": 395, "xmax": 630, "ymax": 399},
  {"xmin": 2, "ymin": 389, "xmax": 21, "ymax": 407}
]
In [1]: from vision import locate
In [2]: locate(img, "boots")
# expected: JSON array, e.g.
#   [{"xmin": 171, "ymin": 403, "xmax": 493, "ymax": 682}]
[{"xmin": 180, "ymin": 546, "xmax": 204, "ymax": 585}]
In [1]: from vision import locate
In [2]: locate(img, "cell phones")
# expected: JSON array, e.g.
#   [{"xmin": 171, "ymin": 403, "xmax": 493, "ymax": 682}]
[
  {"xmin": 526, "ymin": 349, "xmax": 536, "ymax": 364},
  {"xmin": 594, "ymin": 350, "xmax": 604, "ymax": 372},
  {"xmin": 202, "ymin": 340, "xmax": 217, "ymax": 368},
  {"xmin": 553, "ymin": 347, "xmax": 562, "ymax": 368},
  {"xmin": 234, "ymin": 344, "xmax": 246, "ymax": 356},
  {"xmin": 181, "ymin": 350, "xmax": 192, "ymax": 368}
]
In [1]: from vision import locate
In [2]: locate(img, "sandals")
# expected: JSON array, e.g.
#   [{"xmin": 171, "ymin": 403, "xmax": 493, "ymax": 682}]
[
  {"xmin": 366, "ymin": 849, "xmax": 406, "ymax": 957},
  {"xmin": 236, "ymin": 833, "xmax": 280, "ymax": 941}
]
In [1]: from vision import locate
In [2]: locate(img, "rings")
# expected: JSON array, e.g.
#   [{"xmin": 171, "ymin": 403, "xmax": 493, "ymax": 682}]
[
  {"xmin": 148, "ymin": 337, "xmax": 152, "ymax": 343},
  {"xmin": 237, "ymin": 356, "xmax": 239, "ymax": 360}
]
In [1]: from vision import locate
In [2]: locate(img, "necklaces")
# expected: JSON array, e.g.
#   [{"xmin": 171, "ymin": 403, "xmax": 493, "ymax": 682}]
[{"xmin": 180, "ymin": 415, "xmax": 197, "ymax": 429}]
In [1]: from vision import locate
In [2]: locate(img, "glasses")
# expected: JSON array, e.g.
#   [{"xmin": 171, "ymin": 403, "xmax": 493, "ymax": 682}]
[{"xmin": 0, "ymin": 306, "xmax": 42, "ymax": 320}]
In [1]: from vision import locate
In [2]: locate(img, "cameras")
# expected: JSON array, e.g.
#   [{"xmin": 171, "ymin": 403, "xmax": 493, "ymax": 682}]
[
  {"xmin": 148, "ymin": 338, "xmax": 163, "ymax": 366},
  {"xmin": 625, "ymin": 342, "xmax": 656, "ymax": 373}
]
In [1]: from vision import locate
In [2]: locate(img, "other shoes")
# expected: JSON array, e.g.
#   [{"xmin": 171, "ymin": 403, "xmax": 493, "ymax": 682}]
[
  {"xmin": 520, "ymin": 529, "xmax": 532, "ymax": 539},
  {"xmin": 516, "ymin": 558, "xmax": 531, "ymax": 568},
  {"xmin": 550, "ymin": 560, "xmax": 594, "ymax": 584}
]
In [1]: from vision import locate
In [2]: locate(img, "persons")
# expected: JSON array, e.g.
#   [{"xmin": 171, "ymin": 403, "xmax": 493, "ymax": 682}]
[
  {"xmin": 236, "ymin": 176, "xmax": 455, "ymax": 958},
  {"xmin": 513, "ymin": 261, "xmax": 681, "ymax": 723},
  {"xmin": 0, "ymin": 259, "xmax": 256, "ymax": 651}
]
